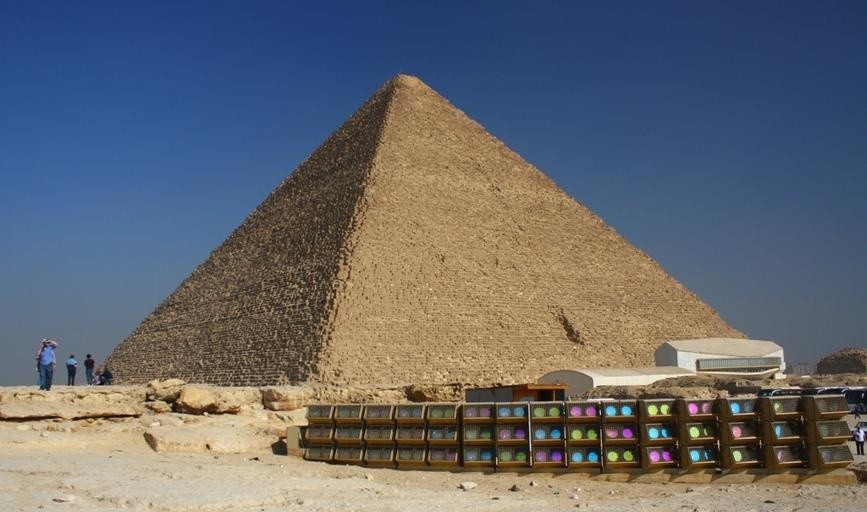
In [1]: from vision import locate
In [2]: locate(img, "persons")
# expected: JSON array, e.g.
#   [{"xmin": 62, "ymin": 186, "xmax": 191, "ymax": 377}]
[
  {"xmin": 94, "ymin": 372, "xmax": 104, "ymax": 385},
  {"xmin": 35, "ymin": 338, "xmax": 58, "ymax": 391},
  {"xmin": 65, "ymin": 353, "xmax": 78, "ymax": 386},
  {"xmin": 99, "ymin": 367, "xmax": 113, "ymax": 385},
  {"xmin": 853, "ymin": 422, "xmax": 866, "ymax": 455},
  {"xmin": 83, "ymin": 353, "xmax": 94, "ymax": 385},
  {"xmin": 37, "ymin": 351, "xmax": 55, "ymax": 389}
]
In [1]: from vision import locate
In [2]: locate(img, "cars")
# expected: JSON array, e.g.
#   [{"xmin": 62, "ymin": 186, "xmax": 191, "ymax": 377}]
[{"xmin": 850, "ymin": 419, "xmax": 867, "ymax": 439}]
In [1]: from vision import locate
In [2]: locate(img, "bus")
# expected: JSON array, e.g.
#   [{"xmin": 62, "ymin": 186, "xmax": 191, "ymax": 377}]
[{"xmin": 758, "ymin": 386, "xmax": 867, "ymax": 413}]
